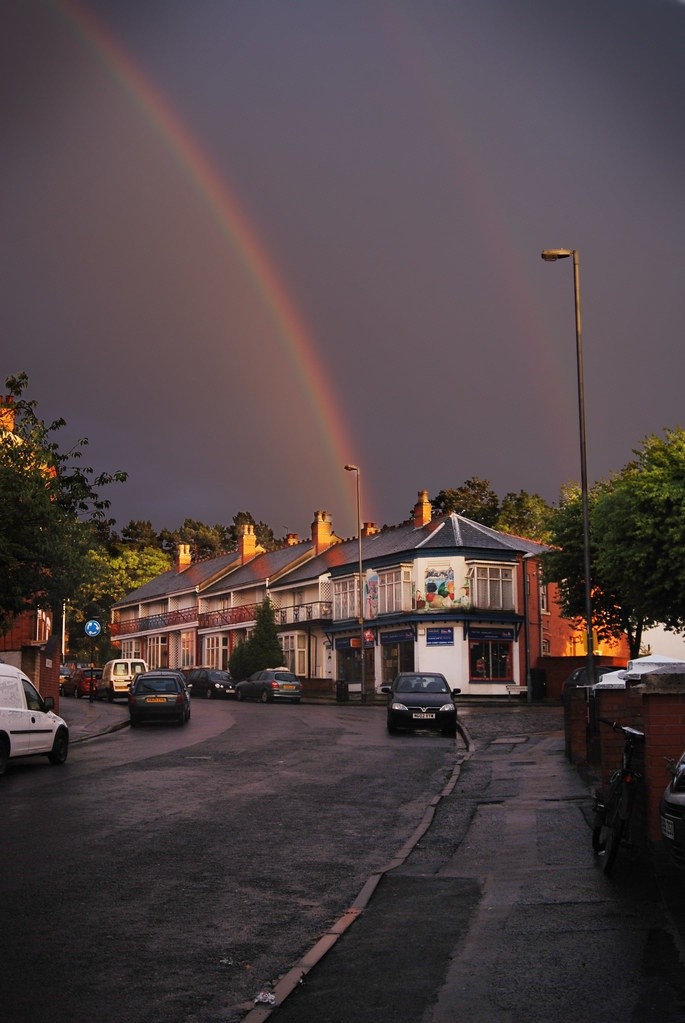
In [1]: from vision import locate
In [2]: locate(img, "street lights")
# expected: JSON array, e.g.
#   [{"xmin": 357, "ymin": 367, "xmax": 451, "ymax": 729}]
[
  {"xmin": 62, "ymin": 597, "xmax": 70, "ymax": 663},
  {"xmin": 539, "ymin": 248, "xmax": 601, "ymax": 768},
  {"xmin": 345, "ymin": 463, "xmax": 368, "ymax": 708}
]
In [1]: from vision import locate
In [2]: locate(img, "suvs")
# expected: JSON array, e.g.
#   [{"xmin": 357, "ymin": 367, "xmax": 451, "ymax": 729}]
[
  {"xmin": 127, "ymin": 668, "xmax": 193, "ymax": 727},
  {"xmin": 0, "ymin": 664, "xmax": 70, "ymax": 775}
]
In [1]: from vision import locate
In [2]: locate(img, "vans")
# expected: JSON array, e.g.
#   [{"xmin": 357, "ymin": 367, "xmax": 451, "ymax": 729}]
[{"xmin": 96, "ymin": 659, "xmax": 150, "ymax": 702}]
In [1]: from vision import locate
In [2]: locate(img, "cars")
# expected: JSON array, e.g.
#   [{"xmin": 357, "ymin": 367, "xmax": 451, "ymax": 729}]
[
  {"xmin": 190, "ymin": 667, "xmax": 239, "ymax": 700},
  {"xmin": 234, "ymin": 667, "xmax": 304, "ymax": 703},
  {"xmin": 559, "ymin": 665, "xmax": 628, "ymax": 705},
  {"xmin": 660, "ymin": 751, "xmax": 685, "ymax": 853},
  {"xmin": 382, "ymin": 664, "xmax": 461, "ymax": 738},
  {"xmin": 59, "ymin": 666, "xmax": 103, "ymax": 699}
]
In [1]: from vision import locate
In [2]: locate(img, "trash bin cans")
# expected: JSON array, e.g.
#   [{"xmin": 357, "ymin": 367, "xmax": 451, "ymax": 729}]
[
  {"xmin": 336, "ymin": 679, "xmax": 349, "ymax": 702},
  {"xmin": 530, "ymin": 668, "xmax": 546, "ymax": 703}
]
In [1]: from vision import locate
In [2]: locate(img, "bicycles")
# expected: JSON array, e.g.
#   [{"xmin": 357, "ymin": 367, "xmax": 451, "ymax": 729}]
[{"xmin": 591, "ymin": 719, "xmax": 645, "ymax": 852}]
